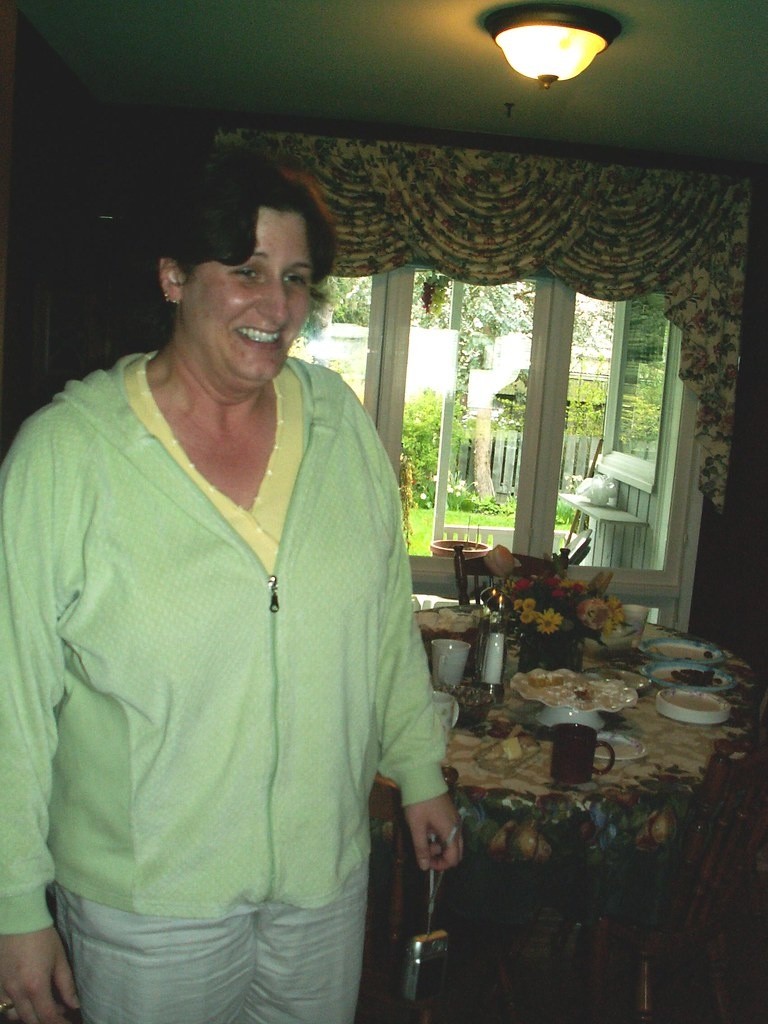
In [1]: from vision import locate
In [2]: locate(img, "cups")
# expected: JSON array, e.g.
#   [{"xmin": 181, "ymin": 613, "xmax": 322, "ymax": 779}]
[
  {"xmin": 433, "ymin": 690, "xmax": 460, "ymax": 750},
  {"xmin": 622, "ymin": 605, "xmax": 650, "ymax": 648},
  {"xmin": 431, "ymin": 639, "xmax": 471, "ymax": 685},
  {"xmin": 550, "ymin": 723, "xmax": 615, "ymax": 785}
]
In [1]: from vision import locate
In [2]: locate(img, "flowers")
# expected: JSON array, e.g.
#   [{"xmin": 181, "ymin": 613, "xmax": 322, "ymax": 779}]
[{"xmin": 480, "ymin": 538, "xmax": 634, "ymax": 647}]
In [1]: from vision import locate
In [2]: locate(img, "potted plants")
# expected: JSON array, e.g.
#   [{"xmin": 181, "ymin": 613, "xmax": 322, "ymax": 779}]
[{"xmin": 430, "ymin": 516, "xmax": 487, "ymax": 557}]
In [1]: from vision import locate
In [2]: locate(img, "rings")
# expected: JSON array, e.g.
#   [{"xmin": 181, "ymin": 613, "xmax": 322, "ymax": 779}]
[{"xmin": 0, "ymin": 1002, "xmax": 15, "ymax": 1010}]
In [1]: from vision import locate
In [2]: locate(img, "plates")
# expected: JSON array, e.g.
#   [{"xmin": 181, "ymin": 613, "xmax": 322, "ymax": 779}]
[
  {"xmin": 655, "ymin": 688, "xmax": 731, "ymax": 724},
  {"xmin": 584, "ymin": 667, "xmax": 653, "ymax": 692},
  {"xmin": 594, "ymin": 732, "xmax": 648, "ymax": 760},
  {"xmin": 639, "ymin": 662, "xmax": 738, "ymax": 692},
  {"xmin": 509, "ymin": 668, "xmax": 638, "ymax": 712},
  {"xmin": 637, "ymin": 638, "xmax": 726, "ymax": 664}
]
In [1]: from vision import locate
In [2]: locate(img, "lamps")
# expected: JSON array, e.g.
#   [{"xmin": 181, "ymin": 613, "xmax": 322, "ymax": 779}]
[{"xmin": 477, "ymin": 5, "xmax": 624, "ymax": 90}]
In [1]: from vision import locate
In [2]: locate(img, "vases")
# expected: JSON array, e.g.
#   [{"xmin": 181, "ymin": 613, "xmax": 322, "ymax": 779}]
[{"xmin": 508, "ymin": 631, "xmax": 586, "ymax": 670}]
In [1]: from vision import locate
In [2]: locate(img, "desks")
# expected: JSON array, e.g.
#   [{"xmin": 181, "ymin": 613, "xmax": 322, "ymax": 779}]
[{"xmin": 371, "ymin": 603, "xmax": 758, "ymax": 1024}]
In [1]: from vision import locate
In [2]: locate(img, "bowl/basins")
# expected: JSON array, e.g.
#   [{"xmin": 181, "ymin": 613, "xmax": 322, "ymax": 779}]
[{"xmin": 434, "ymin": 685, "xmax": 493, "ymax": 728}]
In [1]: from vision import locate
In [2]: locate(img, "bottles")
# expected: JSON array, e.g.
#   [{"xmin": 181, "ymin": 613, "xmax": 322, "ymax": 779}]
[{"xmin": 476, "ymin": 594, "xmax": 508, "ymax": 696}]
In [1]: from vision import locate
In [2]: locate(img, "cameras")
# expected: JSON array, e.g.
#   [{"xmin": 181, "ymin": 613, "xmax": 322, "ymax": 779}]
[{"xmin": 404, "ymin": 930, "xmax": 449, "ymax": 1000}]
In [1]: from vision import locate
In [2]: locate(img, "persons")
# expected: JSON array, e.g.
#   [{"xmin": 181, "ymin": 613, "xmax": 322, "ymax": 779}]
[{"xmin": 1, "ymin": 163, "xmax": 464, "ymax": 1024}]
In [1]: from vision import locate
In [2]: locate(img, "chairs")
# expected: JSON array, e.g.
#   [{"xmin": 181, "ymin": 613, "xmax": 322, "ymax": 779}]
[
  {"xmin": 586, "ymin": 736, "xmax": 768, "ymax": 1021},
  {"xmin": 360, "ymin": 775, "xmax": 518, "ymax": 1019},
  {"xmin": 453, "ymin": 543, "xmax": 572, "ymax": 610},
  {"xmin": 557, "ymin": 530, "xmax": 594, "ymax": 565}
]
